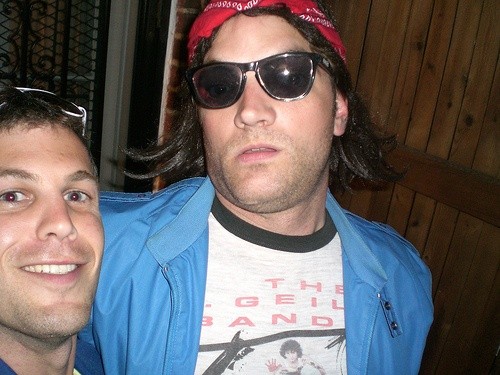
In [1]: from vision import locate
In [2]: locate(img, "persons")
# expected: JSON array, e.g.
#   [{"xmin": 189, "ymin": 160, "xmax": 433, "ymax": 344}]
[
  {"xmin": 0, "ymin": 87, "xmax": 106, "ymax": 374},
  {"xmin": 78, "ymin": 0, "xmax": 434, "ymax": 375}
]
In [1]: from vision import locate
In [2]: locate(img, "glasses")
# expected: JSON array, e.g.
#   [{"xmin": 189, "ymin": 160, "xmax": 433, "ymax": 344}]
[
  {"xmin": 184, "ymin": 51, "xmax": 341, "ymax": 111},
  {"xmin": 0, "ymin": 86, "xmax": 86, "ymax": 137}
]
